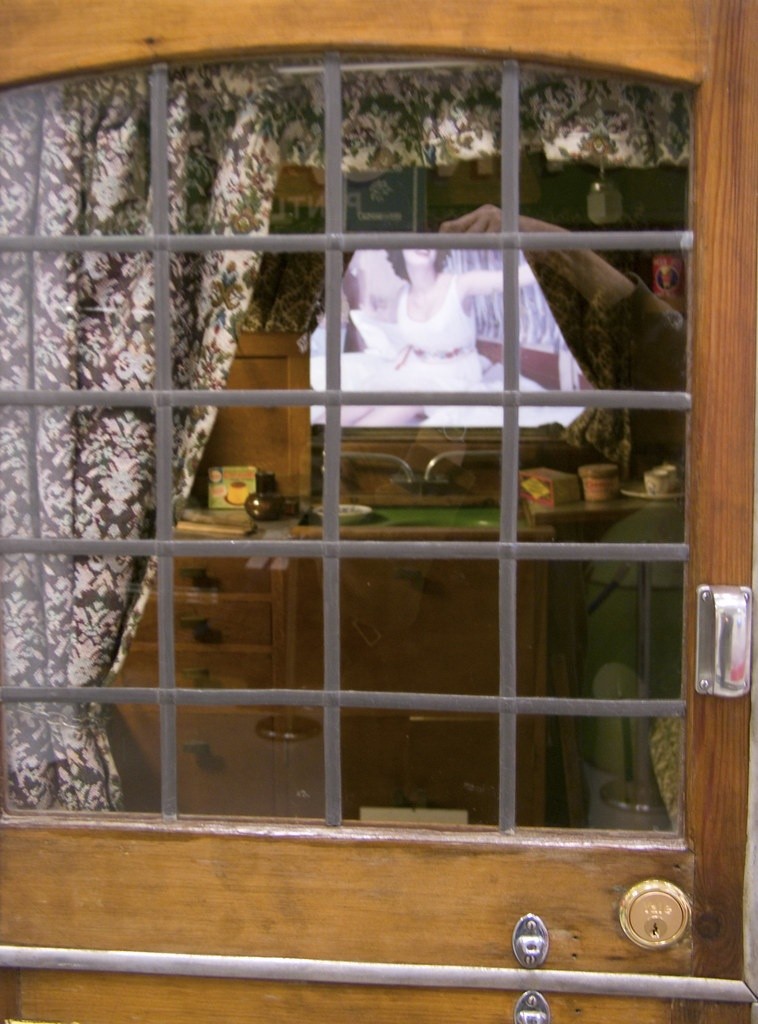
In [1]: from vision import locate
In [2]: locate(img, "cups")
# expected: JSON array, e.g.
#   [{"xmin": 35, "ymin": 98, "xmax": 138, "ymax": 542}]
[{"xmin": 644, "ymin": 465, "xmax": 678, "ymax": 495}]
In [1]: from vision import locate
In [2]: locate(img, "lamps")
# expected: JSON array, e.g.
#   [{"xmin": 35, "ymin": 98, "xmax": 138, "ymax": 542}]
[{"xmin": 584, "ymin": 166, "xmax": 623, "ymax": 226}]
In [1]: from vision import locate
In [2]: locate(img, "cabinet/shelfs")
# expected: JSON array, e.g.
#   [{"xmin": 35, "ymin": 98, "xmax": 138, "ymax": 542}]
[{"xmin": 102, "ymin": 556, "xmax": 290, "ymax": 816}]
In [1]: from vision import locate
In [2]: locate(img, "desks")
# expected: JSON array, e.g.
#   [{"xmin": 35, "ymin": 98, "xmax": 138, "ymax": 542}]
[{"xmin": 523, "ymin": 494, "xmax": 684, "ymax": 815}]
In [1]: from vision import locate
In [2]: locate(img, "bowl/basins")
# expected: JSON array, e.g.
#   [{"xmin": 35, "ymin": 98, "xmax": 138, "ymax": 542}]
[{"xmin": 245, "ymin": 493, "xmax": 285, "ymax": 520}]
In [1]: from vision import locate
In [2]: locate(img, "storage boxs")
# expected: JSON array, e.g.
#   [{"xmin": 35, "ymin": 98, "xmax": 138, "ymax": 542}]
[{"xmin": 207, "ymin": 463, "xmax": 276, "ymax": 511}]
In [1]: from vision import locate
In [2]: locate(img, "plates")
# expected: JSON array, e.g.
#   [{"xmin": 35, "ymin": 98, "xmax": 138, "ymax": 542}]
[
  {"xmin": 313, "ymin": 504, "xmax": 372, "ymax": 524},
  {"xmin": 620, "ymin": 482, "xmax": 682, "ymax": 499}
]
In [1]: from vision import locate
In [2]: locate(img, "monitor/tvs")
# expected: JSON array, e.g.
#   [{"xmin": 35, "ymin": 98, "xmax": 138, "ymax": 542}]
[{"xmin": 307, "ymin": 243, "xmax": 598, "ymax": 441}]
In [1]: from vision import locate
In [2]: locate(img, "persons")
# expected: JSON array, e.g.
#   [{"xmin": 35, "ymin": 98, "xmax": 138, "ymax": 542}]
[
  {"xmin": 349, "ymin": 246, "xmax": 536, "ymax": 430},
  {"xmin": 439, "ymin": 202, "xmax": 689, "ymax": 833}
]
